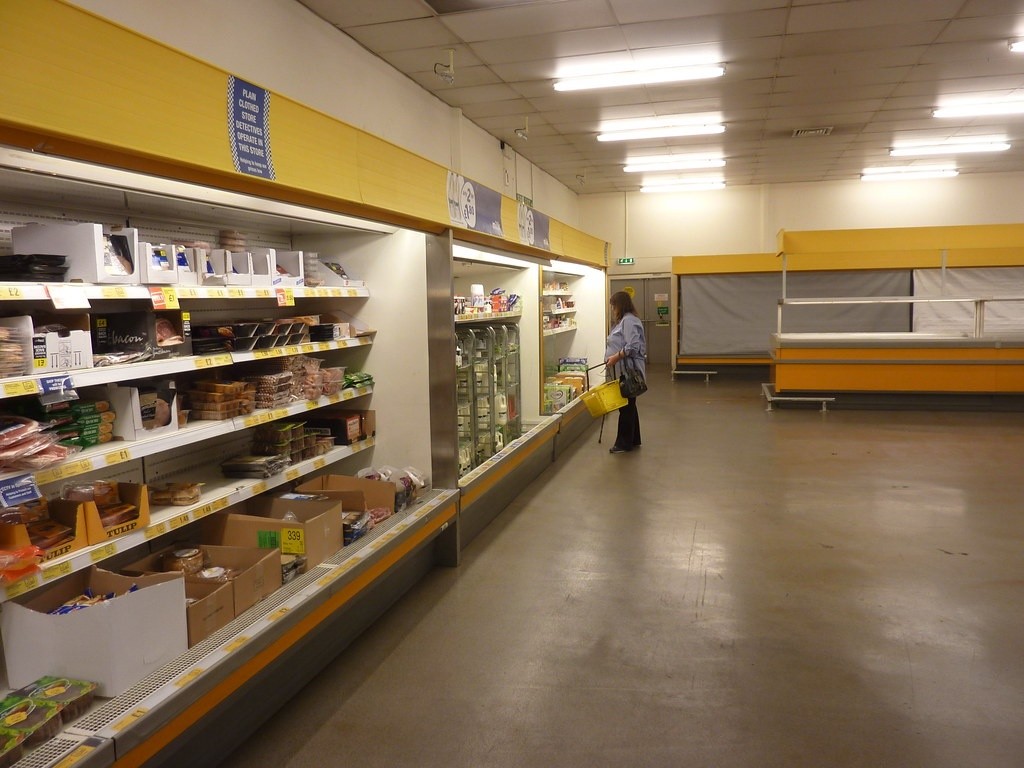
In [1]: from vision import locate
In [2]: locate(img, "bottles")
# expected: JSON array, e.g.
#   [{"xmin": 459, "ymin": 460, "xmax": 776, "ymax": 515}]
[{"xmin": 457, "ymin": 360, "xmax": 507, "ymax": 478}]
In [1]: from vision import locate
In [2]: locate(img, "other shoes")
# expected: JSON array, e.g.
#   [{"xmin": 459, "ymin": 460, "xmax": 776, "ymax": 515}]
[{"xmin": 610, "ymin": 445, "xmax": 629, "ymax": 452}]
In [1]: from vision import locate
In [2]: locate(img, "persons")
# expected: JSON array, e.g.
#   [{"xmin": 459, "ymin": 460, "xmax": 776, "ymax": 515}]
[{"xmin": 604, "ymin": 292, "xmax": 648, "ymax": 454}]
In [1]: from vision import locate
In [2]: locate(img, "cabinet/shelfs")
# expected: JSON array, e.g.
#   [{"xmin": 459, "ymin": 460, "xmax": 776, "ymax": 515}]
[
  {"xmin": 456, "ymin": 310, "xmax": 523, "ymax": 483},
  {"xmin": 542, "ymin": 289, "xmax": 577, "ymax": 337},
  {"xmin": 0, "ymin": 146, "xmax": 378, "ymax": 607}
]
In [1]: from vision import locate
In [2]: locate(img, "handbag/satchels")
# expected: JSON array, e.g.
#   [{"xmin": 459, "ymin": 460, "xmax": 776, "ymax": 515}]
[{"xmin": 619, "ymin": 347, "xmax": 648, "ymax": 397}]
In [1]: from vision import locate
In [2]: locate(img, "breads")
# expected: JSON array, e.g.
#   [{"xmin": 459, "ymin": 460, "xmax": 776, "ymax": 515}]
[{"xmin": 160, "ymin": 545, "xmax": 247, "ymax": 606}]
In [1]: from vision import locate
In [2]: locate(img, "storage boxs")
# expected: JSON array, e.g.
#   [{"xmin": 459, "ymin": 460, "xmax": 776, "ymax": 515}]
[
  {"xmin": 0, "ymin": 312, "xmax": 93, "ymax": 376},
  {"xmin": 84, "ymin": 379, "xmax": 179, "ymax": 441},
  {"xmin": 89, "ymin": 310, "xmax": 193, "ymax": 361},
  {"xmin": 0, "ymin": 476, "xmax": 396, "ymax": 699},
  {"xmin": 11, "ymin": 223, "xmax": 304, "ymax": 285}
]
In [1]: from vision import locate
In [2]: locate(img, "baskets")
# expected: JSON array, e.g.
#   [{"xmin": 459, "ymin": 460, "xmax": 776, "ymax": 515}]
[{"xmin": 580, "ymin": 362, "xmax": 629, "ymax": 418}]
